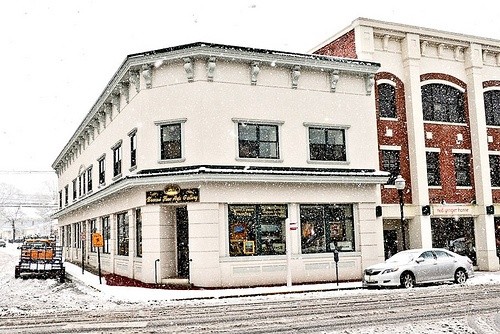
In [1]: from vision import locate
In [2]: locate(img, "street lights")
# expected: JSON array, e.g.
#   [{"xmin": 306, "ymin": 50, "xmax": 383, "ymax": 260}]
[{"xmin": 395, "ymin": 174, "xmax": 406, "ymax": 252}]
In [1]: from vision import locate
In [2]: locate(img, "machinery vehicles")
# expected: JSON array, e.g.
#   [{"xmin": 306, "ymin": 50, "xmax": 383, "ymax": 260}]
[{"xmin": 14, "ymin": 233, "xmax": 65, "ymax": 283}]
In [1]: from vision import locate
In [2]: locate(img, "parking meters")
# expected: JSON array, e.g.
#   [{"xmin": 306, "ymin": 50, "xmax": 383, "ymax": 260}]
[{"xmin": 333, "ymin": 250, "xmax": 339, "ymax": 286}]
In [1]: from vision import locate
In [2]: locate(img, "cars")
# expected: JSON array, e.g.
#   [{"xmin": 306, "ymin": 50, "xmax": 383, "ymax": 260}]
[
  {"xmin": 362, "ymin": 248, "xmax": 475, "ymax": 290},
  {"xmin": 0, "ymin": 239, "xmax": 6, "ymax": 247}
]
